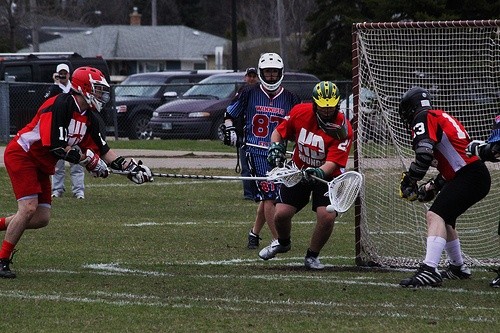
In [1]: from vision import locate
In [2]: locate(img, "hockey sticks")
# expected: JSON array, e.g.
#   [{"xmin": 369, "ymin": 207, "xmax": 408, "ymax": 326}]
[
  {"xmin": 281, "ymin": 161, "xmax": 365, "ymax": 214},
  {"xmin": 110, "ymin": 167, "xmax": 303, "ymax": 189},
  {"xmin": 240, "ymin": 141, "xmax": 293, "ymax": 154}
]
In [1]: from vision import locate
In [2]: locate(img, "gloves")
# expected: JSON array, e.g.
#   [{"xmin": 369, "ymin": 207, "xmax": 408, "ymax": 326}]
[
  {"xmin": 125, "ymin": 158, "xmax": 154, "ymax": 185},
  {"xmin": 399, "ymin": 171, "xmax": 419, "ymax": 201},
  {"xmin": 267, "ymin": 142, "xmax": 287, "ymax": 167},
  {"xmin": 80, "ymin": 149, "xmax": 110, "ymax": 178},
  {"xmin": 466, "ymin": 139, "xmax": 488, "ymax": 156},
  {"xmin": 418, "ymin": 178, "xmax": 440, "ymax": 201},
  {"xmin": 297, "ymin": 167, "xmax": 325, "ymax": 186},
  {"xmin": 218, "ymin": 118, "xmax": 246, "ymax": 149}
]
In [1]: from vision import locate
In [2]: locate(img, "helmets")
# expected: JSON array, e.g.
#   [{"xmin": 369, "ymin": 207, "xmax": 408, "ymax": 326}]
[
  {"xmin": 399, "ymin": 87, "xmax": 432, "ymax": 124},
  {"xmin": 312, "ymin": 81, "xmax": 340, "ymax": 122},
  {"xmin": 257, "ymin": 53, "xmax": 284, "ymax": 92},
  {"xmin": 72, "ymin": 67, "xmax": 110, "ymax": 112}
]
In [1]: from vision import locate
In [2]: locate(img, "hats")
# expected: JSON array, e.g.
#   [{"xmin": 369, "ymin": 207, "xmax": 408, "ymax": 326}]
[
  {"xmin": 57, "ymin": 64, "xmax": 69, "ymax": 72},
  {"xmin": 245, "ymin": 68, "xmax": 257, "ymax": 76}
]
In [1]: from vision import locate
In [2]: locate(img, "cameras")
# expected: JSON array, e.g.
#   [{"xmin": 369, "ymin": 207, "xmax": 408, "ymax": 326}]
[{"xmin": 55, "ymin": 75, "xmax": 66, "ymax": 79}]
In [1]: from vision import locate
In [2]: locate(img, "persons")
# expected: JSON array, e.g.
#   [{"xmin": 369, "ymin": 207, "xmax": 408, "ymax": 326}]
[
  {"xmin": 259, "ymin": 81, "xmax": 353, "ymax": 271},
  {"xmin": 0, "ymin": 67, "xmax": 154, "ymax": 279},
  {"xmin": 466, "ymin": 116, "xmax": 500, "ymax": 162},
  {"xmin": 222, "ymin": 52, "xmax": 303, "ymax": 249},
  {"xmin": 232, "ymin": 67, "xmax": 261, "ymax": 202},
  {"xmin": 398, "ymin": 86, "xmax": 492, "ymax": 289},
  {"xmin": 44, "ymin": 63, "xmax": 86, "ymax": 199}
]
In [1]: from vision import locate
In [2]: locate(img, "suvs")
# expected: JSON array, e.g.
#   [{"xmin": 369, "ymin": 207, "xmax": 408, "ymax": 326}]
[{"xmin": 110, "ymin": 69, "xmax": 236, "ymax": 140}]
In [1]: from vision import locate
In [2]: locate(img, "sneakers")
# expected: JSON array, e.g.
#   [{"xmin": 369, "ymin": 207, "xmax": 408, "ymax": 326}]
[
  {"xmin": 439, "ymin": 261, "xmax": 472, "ymax": 281},
  {"xmin": 51, "ymin": 194, "xmax": 61, "ymax": 197},
  {"xmin": 258, "ymin": 239, "xmax": 291, "ymax": 259},
  {"xmin": 249, "ymin": 230, "xmax": 260, "ymax": 248},
  {"xmin": 77, "ymin": 195, "xmax": 84, "ymax": 199},
  {"xmin": 0, "ymin": 258, "xmax": 16, "ymax": 278},
  {"xmin": 305, "ymin": 256, "xmax": 323, "ymax": 269},
  {"xmin": 490, "ymin": 275, "xmax": 500, "ymax": 287},
  {"xmin": 400, "ymin": 263, "xmax": 443, "ymax": 288}
]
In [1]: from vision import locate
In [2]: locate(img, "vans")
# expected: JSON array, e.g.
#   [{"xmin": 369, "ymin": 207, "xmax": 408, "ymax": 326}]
[
  {"xmin": 1, "ymin": 53, "xmax": 111, "ymax": 134},
  {"xmin": 148, "ymin": 71, "xmax": 320, "ymax": 140}
]
